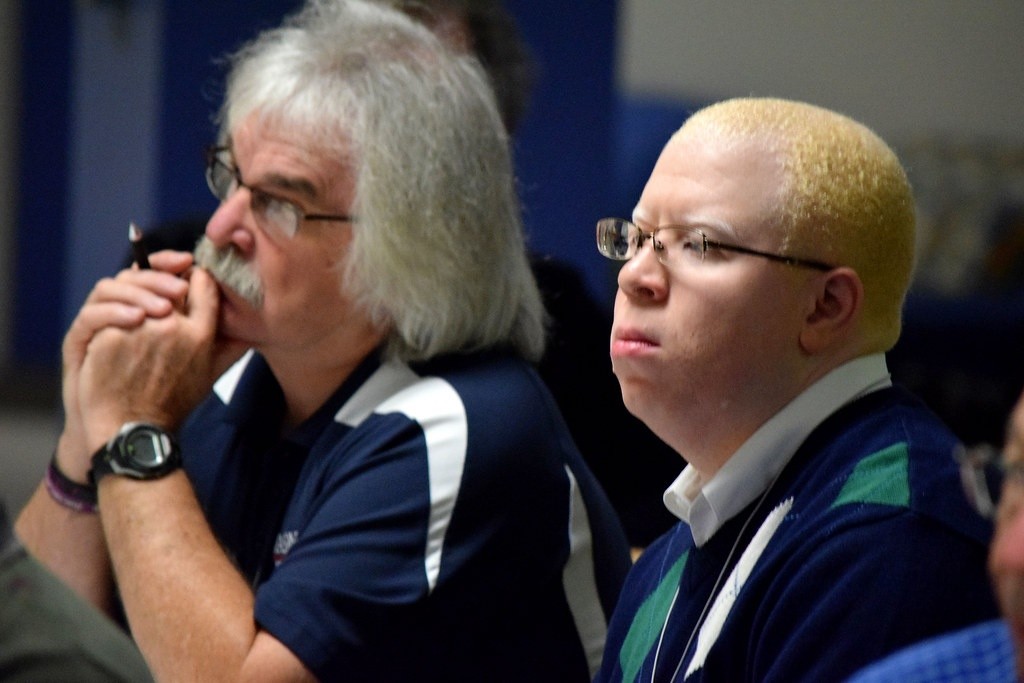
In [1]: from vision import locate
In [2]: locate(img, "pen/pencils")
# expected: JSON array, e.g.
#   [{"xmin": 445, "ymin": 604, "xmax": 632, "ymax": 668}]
[{"xmin": 127, "ymin": 221, "xmax": 151, "ymax": 271}]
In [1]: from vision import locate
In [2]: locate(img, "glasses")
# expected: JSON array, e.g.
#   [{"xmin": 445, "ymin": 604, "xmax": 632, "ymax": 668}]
[
  {"xmin": 596, "ymin": 218, "xmax": 835, "ymax": 272},
  {"xmin": 959, "ymin": 446, "xmax": 1024, "ymax": 521},
  {"xmin": 206, "ymin": 145, "xmax": 352, "ymax": 243}
]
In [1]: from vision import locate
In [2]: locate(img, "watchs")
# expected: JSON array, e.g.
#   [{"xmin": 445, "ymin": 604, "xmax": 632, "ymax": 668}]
[{"xmin": 83, "ymin": 420, "xmax": 188, "ymax": 504}]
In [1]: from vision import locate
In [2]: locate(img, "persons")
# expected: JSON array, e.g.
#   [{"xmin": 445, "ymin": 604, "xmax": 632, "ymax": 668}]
[
  {"xmin": 601, "ymin": 95, "xmax": 1000, "ymax": 683},
  {"xmin": 18, "ymin": 0, "xmax": 637, "ymax": 683},
  {"xmin": 847, "ymin": 390, "xmax": 1024, "ymax": 681},
  {"xmin": 2, "ymin": 509, "xmax": 154, "ymax": 680}
]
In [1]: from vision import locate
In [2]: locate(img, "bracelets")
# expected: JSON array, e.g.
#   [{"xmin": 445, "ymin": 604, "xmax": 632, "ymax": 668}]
[{"xmin": 47, "ymin": 446, "xmax": 98, "ymax": 515}]
